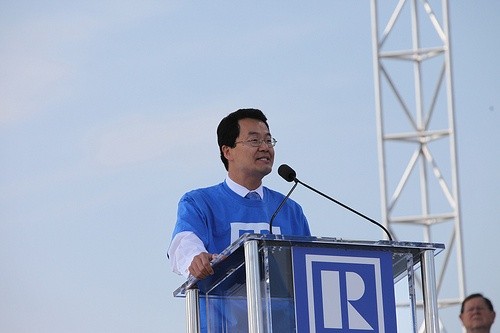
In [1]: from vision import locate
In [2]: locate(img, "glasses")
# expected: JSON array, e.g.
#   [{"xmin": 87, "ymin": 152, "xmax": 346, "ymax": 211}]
[{"xmin": 234, "ymin": 136, "xmax": 277, "ymax": 148}]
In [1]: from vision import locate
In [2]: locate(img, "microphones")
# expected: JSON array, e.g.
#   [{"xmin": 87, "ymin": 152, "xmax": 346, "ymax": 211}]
[{"xmin": 271, "ymin": 164, "xmax": 392, "ymax": 243}]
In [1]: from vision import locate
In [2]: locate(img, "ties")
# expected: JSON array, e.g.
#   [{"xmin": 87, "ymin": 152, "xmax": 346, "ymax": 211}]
[{"xmin": 246, "ymin": 192, "xmax": 261, "ymax": 201}]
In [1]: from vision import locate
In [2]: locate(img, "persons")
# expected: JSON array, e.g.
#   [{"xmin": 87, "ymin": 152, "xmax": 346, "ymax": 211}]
[
  {"xmin": 167, "ymin": 107, "xmax": 311, "ymax": 333},
  {"xmin": 459, "ymin": 293, "xmax": 496, "ymax": 333}
]
